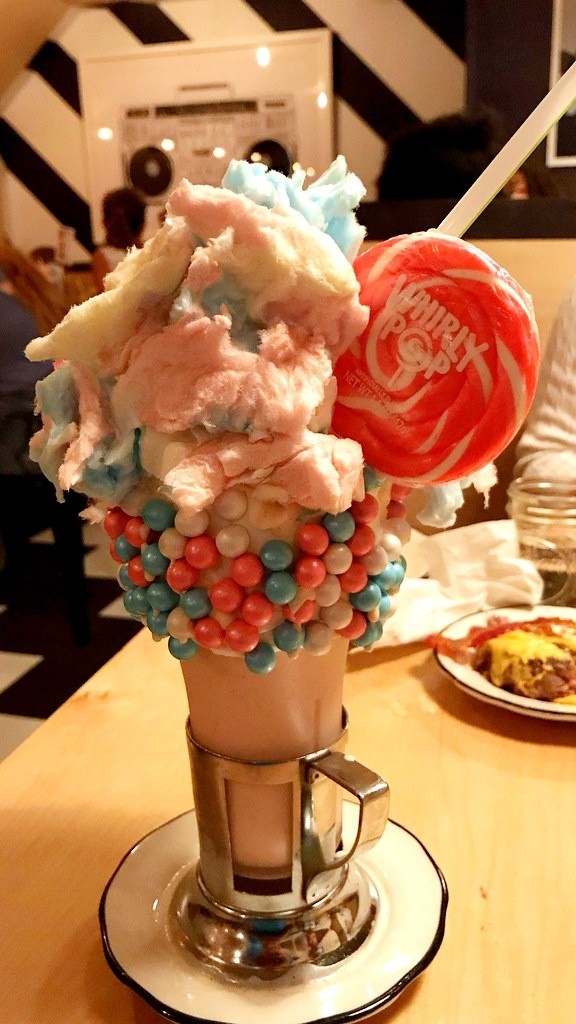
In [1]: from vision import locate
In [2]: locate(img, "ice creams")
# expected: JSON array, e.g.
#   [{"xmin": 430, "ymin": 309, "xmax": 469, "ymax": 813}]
[{"xmin": 20, "ymin": 153, "xmax": 412, "ymax": 918}]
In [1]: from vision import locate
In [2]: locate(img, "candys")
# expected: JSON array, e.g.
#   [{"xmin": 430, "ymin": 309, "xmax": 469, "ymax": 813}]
[{"xmin": 331, "ymin": 229, "xmax": 542, "ymax": 490}]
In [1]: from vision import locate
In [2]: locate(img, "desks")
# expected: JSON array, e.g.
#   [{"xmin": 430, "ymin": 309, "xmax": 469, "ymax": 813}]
[{"xmin": 0, "ymin": 627, "xmax": 576, "ymax": 1024}]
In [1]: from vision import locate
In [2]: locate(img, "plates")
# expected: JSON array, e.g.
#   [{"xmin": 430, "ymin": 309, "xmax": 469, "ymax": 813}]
[
  {"xmin": 99, "ymin": 799, "xmax": 450, "ymax": 1024},
  {"xmin": 434, "ymin": 605, "xmax": 576, "ymax": 722}
]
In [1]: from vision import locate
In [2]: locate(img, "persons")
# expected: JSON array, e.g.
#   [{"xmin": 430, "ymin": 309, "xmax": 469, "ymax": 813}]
[
  {"xmin": 513, "ymin": 291, "xmax": 576, "ymax": 491},
  {"xmin": 377, "ymin": 104, "xmax": 558, "ymax": 201},
  {"xmin": 92, "ymin": 188, "xmax": 146, "ymax": 268},
  {"xmin": 0, "ymin": 233, "xmax": 72, "ymax": 397}
]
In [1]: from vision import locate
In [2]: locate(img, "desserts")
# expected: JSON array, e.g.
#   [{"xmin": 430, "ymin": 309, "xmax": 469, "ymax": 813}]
[{"xmin": 422, "ymin": 616, "xmax": 576, "ymax": 708}]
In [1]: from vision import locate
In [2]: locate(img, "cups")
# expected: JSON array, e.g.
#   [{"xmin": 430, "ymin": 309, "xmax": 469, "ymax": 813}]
[
  {"xmin": 508, "ymin": 475, "xmax": 576, "ymax": 607},
  {"xmin": 172, "ymin": 705, "xmax": 390, "ymax": 985}
]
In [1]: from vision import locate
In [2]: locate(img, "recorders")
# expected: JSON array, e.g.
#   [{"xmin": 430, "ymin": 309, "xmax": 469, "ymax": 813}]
[{"xmin": 119, "ymin": 82, "xmax": 299, "ymax": 208}]
